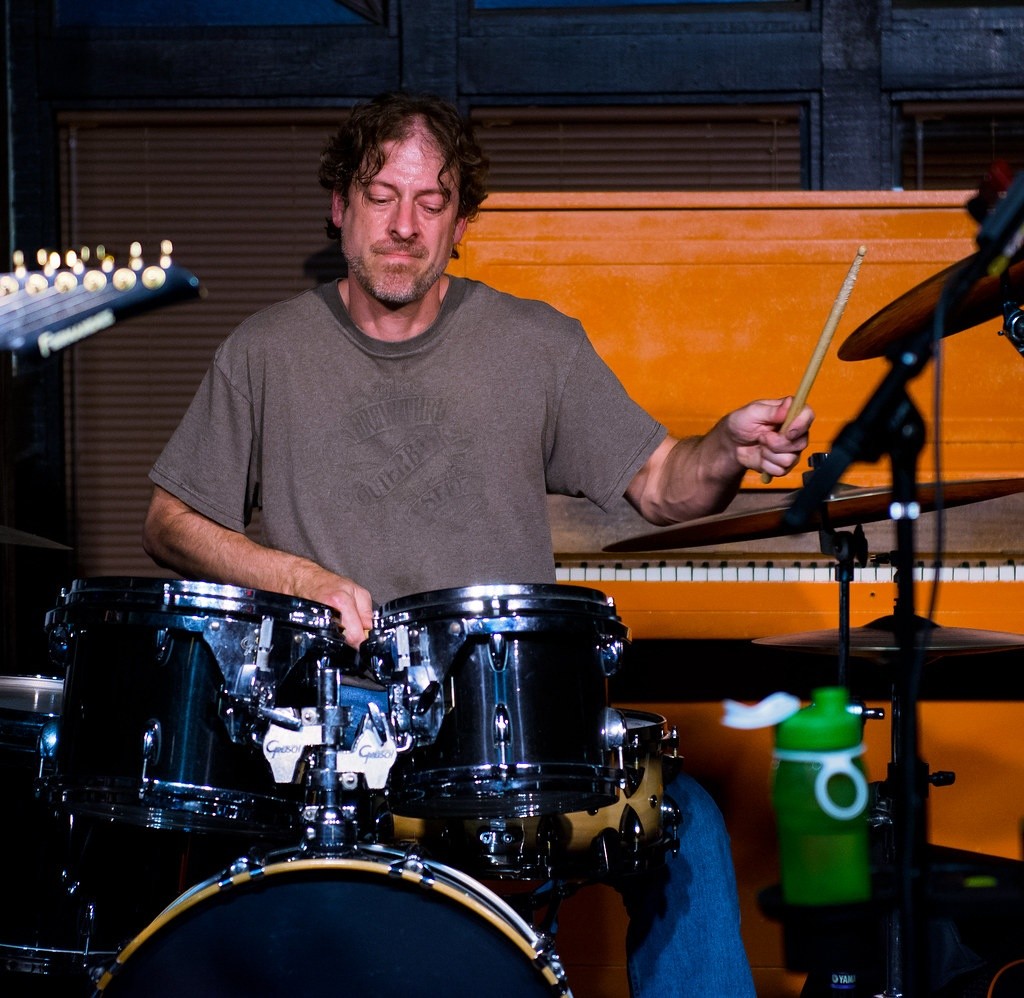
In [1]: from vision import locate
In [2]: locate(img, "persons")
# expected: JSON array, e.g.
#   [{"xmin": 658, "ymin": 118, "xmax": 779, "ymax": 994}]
[{"xmin": 140, "ymin": 90, "xmax": 815, "ymax": 998}]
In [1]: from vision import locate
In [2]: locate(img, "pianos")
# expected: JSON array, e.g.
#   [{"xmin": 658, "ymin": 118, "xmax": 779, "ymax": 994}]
[{"xmin": 445, "ymin": 185, "xmax": 1024, "ymax": 998}]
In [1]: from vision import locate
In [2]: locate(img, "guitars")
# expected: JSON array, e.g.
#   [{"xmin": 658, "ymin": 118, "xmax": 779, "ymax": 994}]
[{"xmin": 0, "ymin": 238, "xmax": 207, "ymax": 387}]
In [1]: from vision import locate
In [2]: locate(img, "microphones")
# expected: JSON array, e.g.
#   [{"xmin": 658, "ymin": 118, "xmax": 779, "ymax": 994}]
[{"xmin": 938, "ymin": 171, "xmax": 1024, "ymax": 323}]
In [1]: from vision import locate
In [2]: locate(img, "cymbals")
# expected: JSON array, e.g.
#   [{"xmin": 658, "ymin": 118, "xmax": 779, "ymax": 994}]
[
  {"xmin": 601, "ymin": 479, "xmax": 1024, "ymax": 554},
  {"xmin": 750, "ymin": 621, "xmax": 1024, "ymax": 666},
  {"xmin": 837, "ymin": 247, "xmax": 1024, "ymax": 363}
]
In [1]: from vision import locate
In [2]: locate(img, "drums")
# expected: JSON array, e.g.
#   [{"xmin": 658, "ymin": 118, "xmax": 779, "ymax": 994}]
[
  {"xmin": 390, "ymin": 706, "xmax": 686, "ymax": 910},
  {"xmin": 0, "ymin": 673, "xmax": 137, "ymax": 975},
  {"xmin": 87, "ymin": 845, "xmax": 581, "ymax": 998},
  {"xmin": 360, "ymin": 579, "xmax": 632, "ymax": 820},
  {"xmin": 55, "ymin": 573, "xmax": 348, "ymax": 841}
]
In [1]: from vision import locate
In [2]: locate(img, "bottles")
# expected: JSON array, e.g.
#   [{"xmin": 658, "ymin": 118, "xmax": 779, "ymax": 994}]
[{"xmin": 767, "ymin": 687, "xmax": 873, "ymax": 904}]
